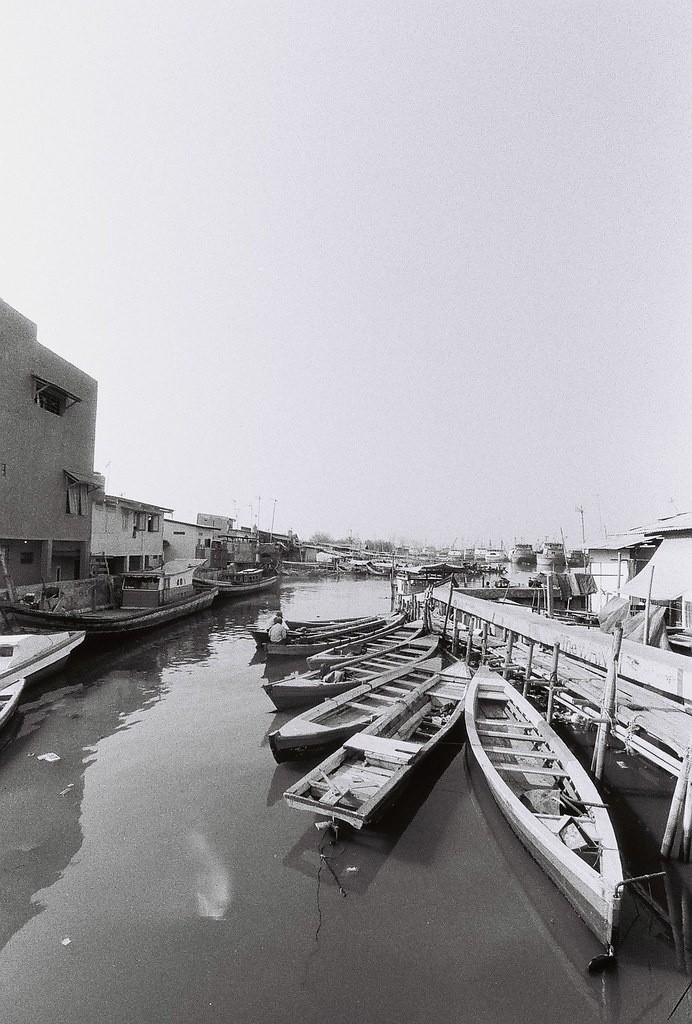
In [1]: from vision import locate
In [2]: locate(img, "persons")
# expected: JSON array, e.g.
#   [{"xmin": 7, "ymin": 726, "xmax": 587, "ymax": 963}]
[
  {"xmin": 266, "ymin": 612, "xmax": 293, "ymax": 644},
  {"xmin": 529, "ymin": 577, "xmax": 533, "ymax": 587}
]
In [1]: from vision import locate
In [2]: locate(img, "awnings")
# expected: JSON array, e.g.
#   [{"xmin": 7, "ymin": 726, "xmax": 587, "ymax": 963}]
[
  {"xmin": 63, "ymin": 469, "xmax": 103, "ymax": 492},
  {"xmin": 31, "ymin": 371, "xmax": 82, "ymax": 409}
]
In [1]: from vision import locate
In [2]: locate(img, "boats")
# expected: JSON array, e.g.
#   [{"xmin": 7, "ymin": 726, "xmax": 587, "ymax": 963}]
[
  {"xmin": 0, "ymin": 631, "xmax": 87, "ymax": 733},
  {"xmin": 464, "ymin": 664, "xmax": 668, "ymax": 953},
  {"xmin": 248, "ymin": 611, "xmax": 439, "ymax": 712},
  {"xmin": 268, "ymin": 654, "xmax": 443, "ymax": 764},
  {"xmin": 316, "ymin": 503, "xmax": 589, "ymax": 586},
  {"xmin": 0, "ymin": 535, "xmax": 285, "ymax": 643},
  {"xmin": 283, "ymin": 660, "xmax": 475, "ymax": 830}
]
